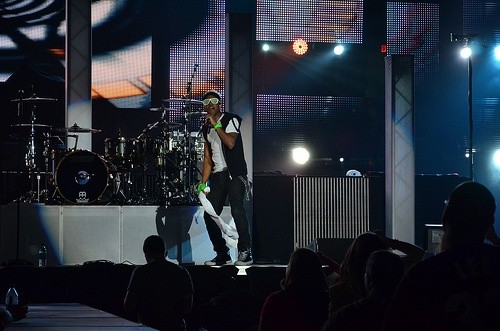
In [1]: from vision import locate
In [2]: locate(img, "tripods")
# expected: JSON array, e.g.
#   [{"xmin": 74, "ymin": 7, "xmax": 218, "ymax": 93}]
[{"xmin": 11, "ymin": 100, "xmax": 204, "ymax": 207}]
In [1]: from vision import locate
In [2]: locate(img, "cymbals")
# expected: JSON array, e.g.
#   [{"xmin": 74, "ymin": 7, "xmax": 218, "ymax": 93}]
[
  {"xmin": 10, "ymin": 123, "xmax": 53, "ymax": 128},
  {"xmin": 10, "ymin": 96, "xmax": 58, "ymax": 103},
  {"xmin": 182, "ymin": 111, "xmax": 208, "ymax": 116},
  {"xmin": 149, "ymin": 107, "xmax": 178, "ymax": 112},
  {"xmin": 161, "ymin": 98, "xmax": 203, "ymax": 104},
  {"xmin": 49, "ymin": 126, "xmax": 102, "ymax": 133}
]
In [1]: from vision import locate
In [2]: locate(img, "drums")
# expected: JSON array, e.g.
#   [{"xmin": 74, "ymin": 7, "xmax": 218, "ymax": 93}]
[
  {"xmin": 137, "ymin": 146, "xmax": 165, "ymax": 174},
  {"xmin": 104, "ymin": 136, "xmax": 136, "ymax": 158},
  {"xmin": 55, "ymin": 149, "xmax": 120, "ymax": 205},
  {"xmin": 147, "ymin": 121, "xmax": 182, "ymax": 146},
  {"xmin": 165, "ymin": 130, "xmax": 205, "ymax": 155}
]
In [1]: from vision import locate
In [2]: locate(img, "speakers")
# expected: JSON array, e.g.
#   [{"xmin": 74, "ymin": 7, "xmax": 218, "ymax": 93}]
[{"xmin": 246, "ymin": 266, "xmax": 287, "ymax": 277}]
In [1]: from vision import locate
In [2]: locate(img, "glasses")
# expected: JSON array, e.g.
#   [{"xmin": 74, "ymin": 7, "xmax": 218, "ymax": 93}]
[{"xmin": 202, "ymin": 98, "xmax": 221, "ymax": 106}]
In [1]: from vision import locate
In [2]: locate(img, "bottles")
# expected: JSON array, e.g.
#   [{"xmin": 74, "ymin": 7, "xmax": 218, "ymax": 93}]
[{"xmin": 38, "ymin": 246, "xmax": 47, "ymax": 267}]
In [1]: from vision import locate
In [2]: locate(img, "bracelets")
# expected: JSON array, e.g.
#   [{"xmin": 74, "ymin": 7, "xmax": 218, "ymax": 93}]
[
  {"xmin": 392, "ymin": 238, "xmax": 399, "ymax": 250},
  {"xmin": 214, "ymin": 120, "xmax": 222, "ymax": 129}
]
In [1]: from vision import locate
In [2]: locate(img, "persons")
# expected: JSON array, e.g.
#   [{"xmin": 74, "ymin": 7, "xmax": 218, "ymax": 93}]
[
  {"xmin": 124, "ymin": 235, "xmax": 194, "ymax": 331},
  {"xmin": 196, "ymin": 92, "xmax": 253, "ymax": 265},
  {"xmin": 259, "ymin": 181, "xmax": 500, "ymax": 331}
]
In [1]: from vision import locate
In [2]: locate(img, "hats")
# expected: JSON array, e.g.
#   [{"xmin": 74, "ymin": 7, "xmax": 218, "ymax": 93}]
[{"xmin": 203, "ymin": 90, "xmax": 223, "ymax": 101}]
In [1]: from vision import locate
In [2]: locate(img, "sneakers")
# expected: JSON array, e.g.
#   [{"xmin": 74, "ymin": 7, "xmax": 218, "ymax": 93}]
[
  {"xmin": 204, "ymin": 251, "xmax": 232, "ymax": 265},
  {"xmin": 234, "ymin": 249, "xmax": 253, "ymax": 265}
]
